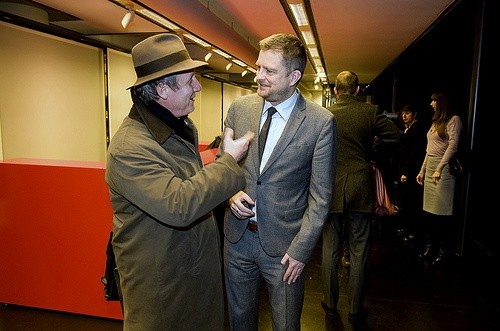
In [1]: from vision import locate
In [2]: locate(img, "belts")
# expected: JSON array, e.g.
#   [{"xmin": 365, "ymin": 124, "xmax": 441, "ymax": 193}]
[{"xmin": 248, "ymin": 220, "xmax": 258, "ymax": 231}]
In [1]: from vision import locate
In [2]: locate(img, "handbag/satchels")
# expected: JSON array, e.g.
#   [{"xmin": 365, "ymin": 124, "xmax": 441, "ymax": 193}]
[
  {"xmin": 448, "ymin": 157, "xmax": 463, "ymax": 174},
  {"xmin": 102, "ymin": 232, "xmax": 120, "ymax": 302}
]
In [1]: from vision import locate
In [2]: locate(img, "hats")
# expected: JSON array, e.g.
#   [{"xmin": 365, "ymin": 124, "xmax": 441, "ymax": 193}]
[{"xmin": 126, "ymin": 33, "xmax": 207, "ymax": 89}]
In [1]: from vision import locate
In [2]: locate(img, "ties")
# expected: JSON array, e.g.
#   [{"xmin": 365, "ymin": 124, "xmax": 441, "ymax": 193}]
[{"xmin": 258, "ymin": 106, "xmax": 277, "ymax": 164}]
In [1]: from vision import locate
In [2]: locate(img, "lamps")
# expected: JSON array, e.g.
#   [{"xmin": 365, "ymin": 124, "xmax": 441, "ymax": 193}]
[
  {"xmin": 225, "ymin": 57, "xmax": 234, "ymax": 72},
  {"xmin": 120, "ymin": 4, "xmax": 135, "ymax": 30},
  {"xmin": 202, "ymin": 47, "xmax": 214, "ymax": 62},
  {"xmin": 241, "ymin": 66, "xmax": 249, "ymax": 77}
]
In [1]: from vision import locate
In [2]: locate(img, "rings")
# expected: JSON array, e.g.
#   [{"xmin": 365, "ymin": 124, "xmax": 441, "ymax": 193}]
[{"xmin": 236, "ymin": 210, "xmax": 238, "ymax": 213}]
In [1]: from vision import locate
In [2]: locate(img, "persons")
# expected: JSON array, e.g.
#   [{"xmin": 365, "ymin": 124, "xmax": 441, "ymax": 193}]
[
  {"xmin": 321, "ymin": 70, "xmax": 402, "ymax": 323},
  {"xmin": 373, "ymin": 105, "xmax": 425, "ymax": 225},
  {"xmin": 104, "ymin": 33, "xmax": 255, "ymax": 331},
  {"xmin": 216, "ymin": 33, "xmax": 336, "ymax": 331},
  {"xmin": 415, "ymin": 88, "xmax": 463, "ymax": 267}
]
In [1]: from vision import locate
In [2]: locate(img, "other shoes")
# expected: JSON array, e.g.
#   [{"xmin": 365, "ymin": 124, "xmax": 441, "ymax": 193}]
[
  {"xmin": 349, "ymin": 306, "xmax": 369, "ymax": 322},
  {"xmin": 432, "ymin": 249, "xmax": 447, "ymax": 265},
  {"xmin": 321, "ymin": 296, "xmax": 336, "ymax": 315},
  {"xmin": 398, "ymin": 228, "xmax": 406, "ymax": 232},
  {"xmin": 420, "ymin": 243, "xmax": 433, "ymax": 258},
  {"xmin": 341, "ymin": 257, "xmax": 350, "ymax": 267},
  {"xmin": 403, "ymin": 234, "xmax": 414, "ymax": 241}
]
